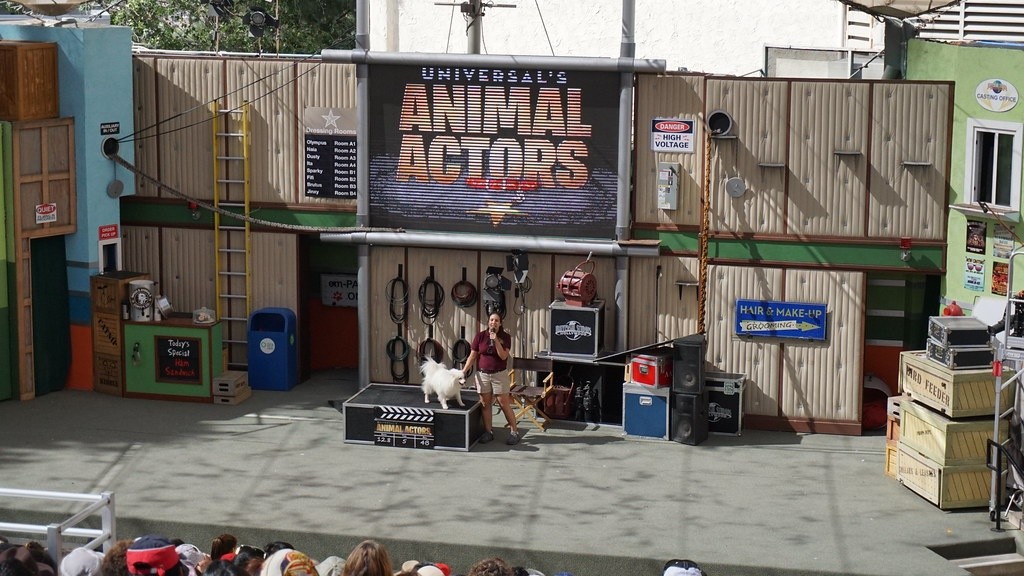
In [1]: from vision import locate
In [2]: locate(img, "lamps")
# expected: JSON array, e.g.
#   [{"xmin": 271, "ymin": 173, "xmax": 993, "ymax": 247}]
[{"xmin": 242, "ymin": 5, "xmax": 279, "ymax": 38}]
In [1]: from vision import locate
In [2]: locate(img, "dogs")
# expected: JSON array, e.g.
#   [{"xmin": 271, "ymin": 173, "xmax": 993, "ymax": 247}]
[{"xmin": 419, "ymin": 356, "xmax": 467, "ymax": 411}]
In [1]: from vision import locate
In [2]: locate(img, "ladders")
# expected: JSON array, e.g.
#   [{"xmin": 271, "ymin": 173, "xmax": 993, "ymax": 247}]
[{"xmin": 211, "ymin": 99, "xmax": 252, "ymax": 368}]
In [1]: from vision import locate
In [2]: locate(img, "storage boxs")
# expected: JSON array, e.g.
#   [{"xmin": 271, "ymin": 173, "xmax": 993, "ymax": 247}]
[
  {"xmin": 89, "ymin": 270, "xmax": 150, "ymax": 406},
  {"xmin": 213, "ymin": 370, "xmax": 253, "ymax": 406},
  {"xmin": 547, "ymin": 299, "xmax": 673, "ymax": 442},
  {"xmin": 886, "ymin": 315, "xmax": 1017, "ymax": 511},
  {"xmin": 704, "ymin": 371, "xmax": 748, "ymax": 437},
  {"xmin": 342, "ymin": 383, "xmax": 484, "ymax": 452}
]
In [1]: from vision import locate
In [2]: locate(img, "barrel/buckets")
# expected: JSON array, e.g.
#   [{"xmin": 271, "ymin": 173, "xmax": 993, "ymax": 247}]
[{"xmin": 128, "ymin": 279, "xmax": 154, "ymax": 322}]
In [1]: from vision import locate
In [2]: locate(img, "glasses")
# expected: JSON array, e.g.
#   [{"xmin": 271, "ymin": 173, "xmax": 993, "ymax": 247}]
[{"xmin": 238, "ymin": 545, "xmax": 266, "ymax": 560}]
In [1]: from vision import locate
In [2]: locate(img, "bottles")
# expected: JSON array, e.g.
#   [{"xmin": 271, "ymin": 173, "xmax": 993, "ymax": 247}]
[{"xmin": 122, "ymin": 304, "xmax": 129, "ymax": 320}]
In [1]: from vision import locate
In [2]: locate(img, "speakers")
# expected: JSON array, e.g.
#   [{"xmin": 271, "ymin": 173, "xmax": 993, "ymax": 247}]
[
  {"xmin": 671, "ymin": 394, "xmax": 709, "ymax": 446},
  {"xmin": 672, "ymin": 334, "xmax": 706, "ymax": 394}
]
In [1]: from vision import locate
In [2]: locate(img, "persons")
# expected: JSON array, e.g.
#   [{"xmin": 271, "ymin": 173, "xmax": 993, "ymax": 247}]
[
  {"xmin": 663, "ymin": 559, "xmax": 702, "ymax": 576},
  {"xmin": 464, "ymin": 311, "xmax": 520, "ymax": 445},
  {"xmin": 0, "ymin": 536, "xmax": 453, "ymax": 576},
  {"xmin": 464, "ymin": 557, "xmax": 545, "ymax": 576}
]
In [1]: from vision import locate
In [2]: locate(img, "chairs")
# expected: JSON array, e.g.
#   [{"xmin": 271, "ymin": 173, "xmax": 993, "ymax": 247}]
[{"xmin": 504, "ymin": 353, "xmax": 555, "ymax": 432}]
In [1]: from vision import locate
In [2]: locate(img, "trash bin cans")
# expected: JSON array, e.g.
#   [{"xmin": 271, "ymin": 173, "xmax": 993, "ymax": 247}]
[{"xmin": 246, "ymin": 307, "xmax": 298, "ymax": 392}]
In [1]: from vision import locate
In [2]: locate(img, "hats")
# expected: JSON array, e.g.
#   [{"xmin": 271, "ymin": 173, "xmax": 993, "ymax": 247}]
[
  {"xmin": 0, "ymin": 547, "xmax": 54, "ymax": 576},
  {"xmin": 60, "ymin": 548, "xmax": 105, "ymax": 576},
  {"xmin": 127, "ymin": 534, "xmax": 179, "ymax": 576},
  {"xmin": 175, "ymin": 544, "xmax": 201, "ymax": 566}
]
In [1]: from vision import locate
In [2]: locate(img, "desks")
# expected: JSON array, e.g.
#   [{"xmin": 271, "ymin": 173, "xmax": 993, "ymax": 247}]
[{"xmin": 121, "ymin": 312, "xmax": 224, "ymax": 404}]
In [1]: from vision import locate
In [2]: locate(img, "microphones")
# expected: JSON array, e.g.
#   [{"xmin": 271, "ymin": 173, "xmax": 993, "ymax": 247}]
[{"xmin": 490, "ymin": 328, "xmax": 496, "ymax": 347}]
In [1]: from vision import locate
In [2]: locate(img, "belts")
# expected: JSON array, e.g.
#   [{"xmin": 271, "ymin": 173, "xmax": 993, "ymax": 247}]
[{"xmin": 477, "ymin": 368, "xmax": 506, "ymax": 374}]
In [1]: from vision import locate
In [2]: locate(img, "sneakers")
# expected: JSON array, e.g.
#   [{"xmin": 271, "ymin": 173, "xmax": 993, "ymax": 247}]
[
  {"xmin": 507, "ymin": 431, "xmax": 520, "ymax": 444},
  {"xmin": 479, "ymin": 430, "xmax": 494, "ymax": 443}
]
